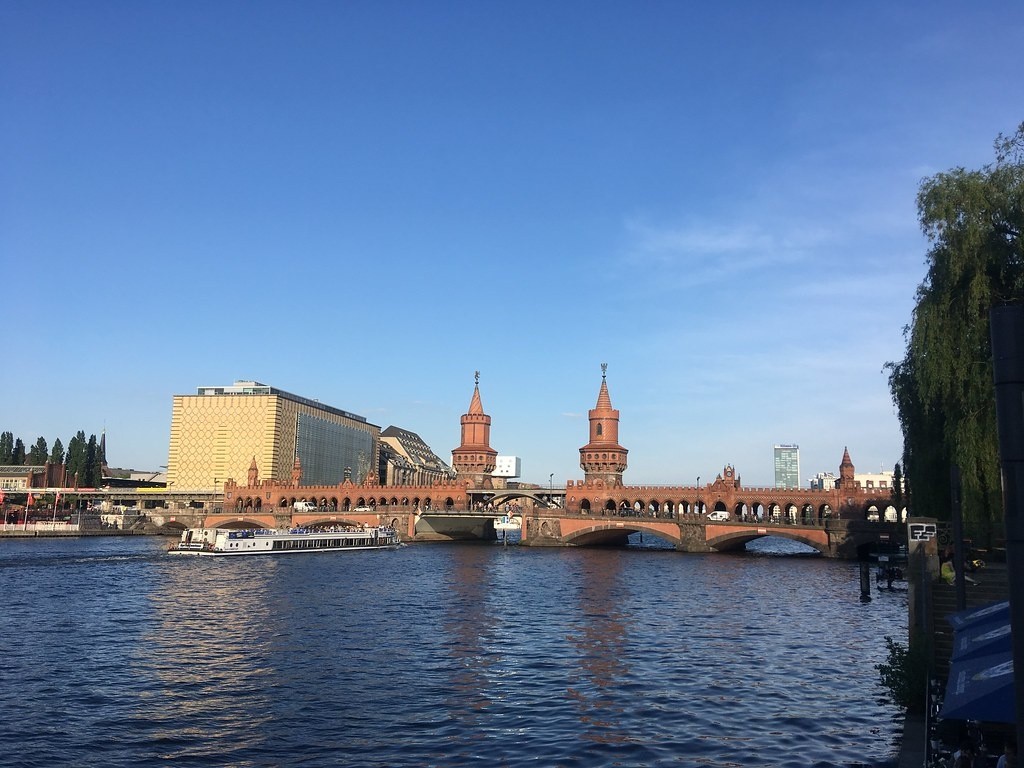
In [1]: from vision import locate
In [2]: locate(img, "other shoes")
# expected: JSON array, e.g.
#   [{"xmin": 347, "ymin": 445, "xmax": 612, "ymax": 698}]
[{"xmin": 974, "ymin": 580, "xmax": 981, "ymax": 586}]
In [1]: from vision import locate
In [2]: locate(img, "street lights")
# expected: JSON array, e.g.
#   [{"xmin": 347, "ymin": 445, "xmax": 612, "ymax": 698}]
[
  {"xmin": 697, "ymin": 476, "xmax": 700, "ymax": 516},
  {"xmin": 550, "ymin": 473, "xmax": 555, "ymax": 505}
]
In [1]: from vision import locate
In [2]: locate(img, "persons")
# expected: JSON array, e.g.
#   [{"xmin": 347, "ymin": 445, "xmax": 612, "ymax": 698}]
[
  {"xmin": 56, "ymin": 499, "xmax": 163, "ymax": 510},
  {"xmin": 308, "ymin": 502, "xmax": 519, "ymax": 533},
  {"xmin": 940, "ymin": 543, "xmax": 982, "ymax": 586},
  {"xmin": 6, "ymin": 512, "xmax": 18, "ymax": 524}
]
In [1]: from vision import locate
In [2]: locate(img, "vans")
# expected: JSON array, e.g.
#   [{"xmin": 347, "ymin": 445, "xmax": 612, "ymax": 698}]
[{"xmin": 707, "ymin": 511, "xmax": 731, "ymax": 522}]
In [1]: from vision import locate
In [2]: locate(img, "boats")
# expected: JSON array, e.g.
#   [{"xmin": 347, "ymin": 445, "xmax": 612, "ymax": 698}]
[{"xmin": 167, "ymin": 522, "xmax": 405, "ymax": 556}]
